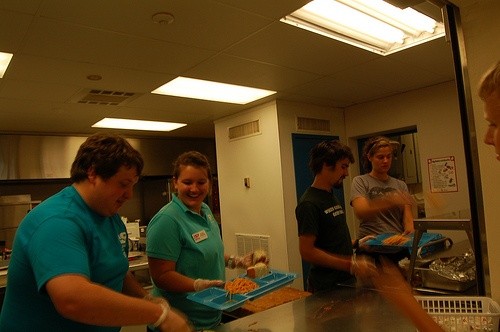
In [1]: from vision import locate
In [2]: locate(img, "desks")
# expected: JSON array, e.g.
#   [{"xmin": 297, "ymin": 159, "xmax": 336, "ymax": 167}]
[
  {"xmin": 0, "ymin": 250, "xmax": 151, "ymax": 290},
  {"xmin": 218, "ymin": 283, "xmax": 313, "ymax": 326}
]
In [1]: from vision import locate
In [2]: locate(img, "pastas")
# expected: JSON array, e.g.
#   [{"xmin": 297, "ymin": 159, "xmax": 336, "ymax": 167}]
[
  {"xmin": 383, "ymin": 236, "xmax": 408, "ymax": 246},
  {"xmin": 224, "ymin": 277, "xmax": 260, "ymax": 299}
]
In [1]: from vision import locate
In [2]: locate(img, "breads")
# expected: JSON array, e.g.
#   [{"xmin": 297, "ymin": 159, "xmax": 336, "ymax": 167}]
[{"xmin": 246, "ymin": 250, "xmax": 270, "ymax": 279}]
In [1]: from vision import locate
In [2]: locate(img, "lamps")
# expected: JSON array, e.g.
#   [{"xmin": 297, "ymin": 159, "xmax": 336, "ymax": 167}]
[{"xmin": 279, "ymin": 0, "xmax": 445, "ymax": 59}]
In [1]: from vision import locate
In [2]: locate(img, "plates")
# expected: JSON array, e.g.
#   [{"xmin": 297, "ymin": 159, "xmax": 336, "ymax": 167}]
[
  {"xmin": 186, "ymin": 269, "xmax": 297, "ymax": 312},
  {"xmin": 363, "ymin": 232, "xmax": 435, "ymax": 248}
]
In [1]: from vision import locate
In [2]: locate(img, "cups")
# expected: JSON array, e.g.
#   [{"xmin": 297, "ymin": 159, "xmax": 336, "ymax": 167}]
[{"xmin": 459, "ymin": 208, "xmax": 469, "ymax": 219}]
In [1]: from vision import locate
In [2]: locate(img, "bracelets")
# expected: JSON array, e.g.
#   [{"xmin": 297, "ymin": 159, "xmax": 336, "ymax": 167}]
[
  {"xmin": 228, "ymin": 255, "xmax": 235, "ymax": 269},
  {"xmin": 354, "ymin": 239, "xmax": 359, "ymax": 248},
  {"xmin": 145, "ymin": 303, "xmax": 167, "ymax": 329}
]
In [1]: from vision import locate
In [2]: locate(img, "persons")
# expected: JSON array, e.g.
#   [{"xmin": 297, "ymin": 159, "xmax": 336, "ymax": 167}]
[
  {"xmin": 295, "ymin": 141, "xmax": 378, "ymax": 332},
  {"xmin": 373, "ymin": 60, "xmax": 500, "ymax": 332},
  {"xmin": 0, "ymin": 133, "xmax": 192, "ymax": 332},
  {"xmin": 349, "ymin": 136, "xmax": 415, "ymax": 288},
  {"xmin": 147, "ymin": 151, "xmax": 268, "ymax": 332}
]
